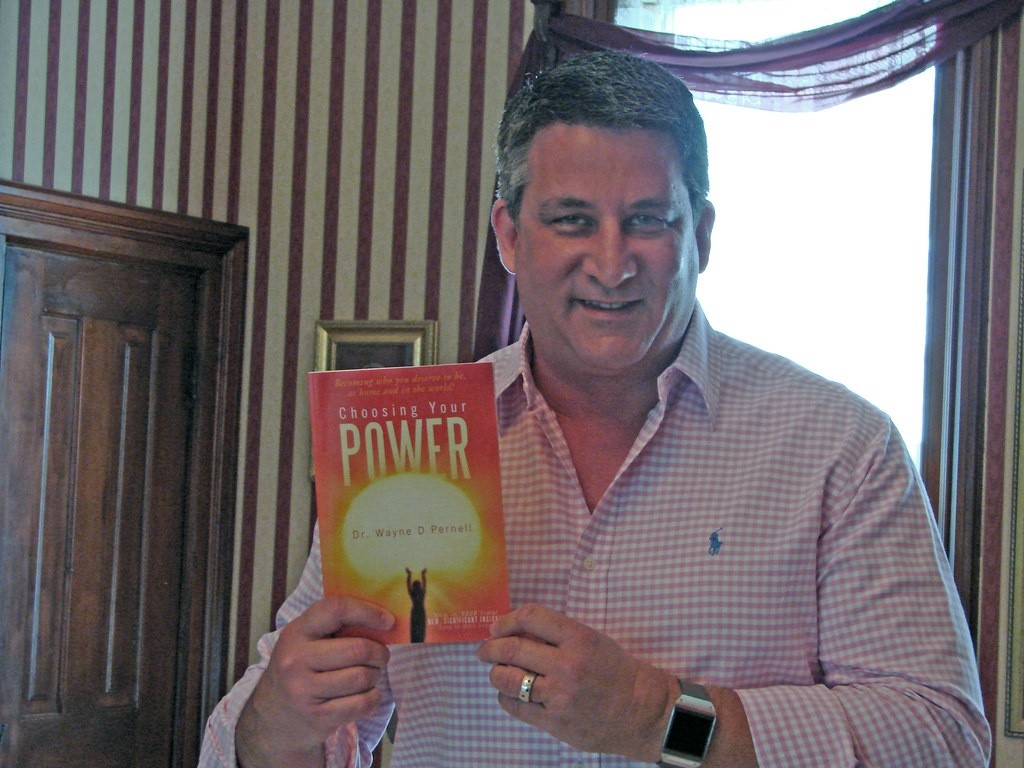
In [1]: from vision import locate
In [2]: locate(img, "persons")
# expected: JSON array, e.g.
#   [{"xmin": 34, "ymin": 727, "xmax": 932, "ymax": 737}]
[{"xmin": 197, "ymin": 50, "xmax": 993, "ymax": 768}]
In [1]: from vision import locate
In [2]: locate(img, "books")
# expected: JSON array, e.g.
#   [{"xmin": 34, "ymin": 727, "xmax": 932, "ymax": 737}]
[{"xmin": 307, "ymin": 361, "xmax": 511, "ymax": 647}]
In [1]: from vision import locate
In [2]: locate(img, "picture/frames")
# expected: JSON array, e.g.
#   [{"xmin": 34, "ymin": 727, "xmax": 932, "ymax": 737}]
[{"xmin": 307, "ymin": 318, "xmax": 441, "ymax": 484}]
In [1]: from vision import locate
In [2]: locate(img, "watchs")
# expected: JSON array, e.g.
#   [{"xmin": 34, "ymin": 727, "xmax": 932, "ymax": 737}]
[{"xmin": 655, "ymin": 678, "xmax": 717, "ymax": 768}]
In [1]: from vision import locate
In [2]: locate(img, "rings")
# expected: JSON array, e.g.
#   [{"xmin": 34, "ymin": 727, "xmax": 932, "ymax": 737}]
[{"xmin": 518, "ymin": 672, "xmax": 538, "ymax": 703}]
